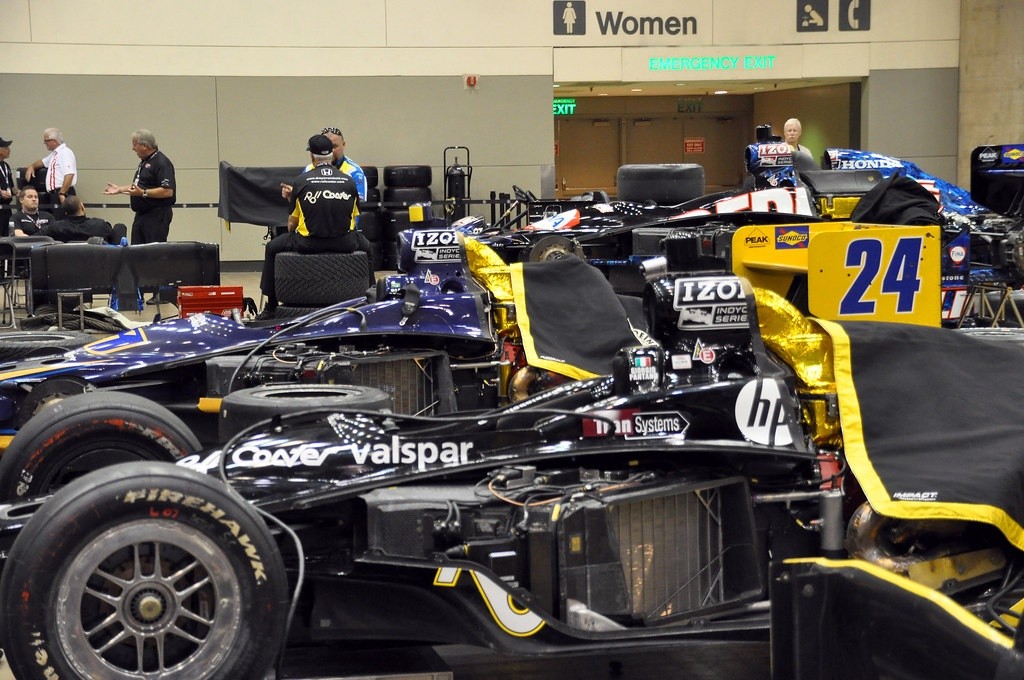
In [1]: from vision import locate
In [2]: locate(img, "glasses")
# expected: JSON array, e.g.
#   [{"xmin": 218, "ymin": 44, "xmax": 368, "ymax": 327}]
[
  {"xmin": 321, "ymin": 127, "xmax": 342, "ymax": 136},
  {"xmin": 44, "ymin": 139, "xmax": 55, "ymax": 142}
]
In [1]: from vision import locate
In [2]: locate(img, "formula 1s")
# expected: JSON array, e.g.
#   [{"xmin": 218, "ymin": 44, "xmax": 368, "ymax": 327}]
[{"xmin": 0, "ymin": 124, "xmax": 1024, "ymax": 680}]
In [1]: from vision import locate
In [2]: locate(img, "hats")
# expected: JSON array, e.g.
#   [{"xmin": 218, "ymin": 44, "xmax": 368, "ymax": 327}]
[
  {"xmin": 0, "ymin": 137, "xmax": 13, "ymax": 147},
  {"xmin": 306, "ymin": 135, "xmax": 333, "ymax": 156}
]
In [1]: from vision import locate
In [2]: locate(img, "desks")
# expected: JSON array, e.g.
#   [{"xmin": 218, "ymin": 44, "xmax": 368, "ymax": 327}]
[
  {"xmin": 31, "ymin": 239, "xmax": 221, "ymax": 321},
  {"xmin": 0, "ymin": 242, "xmax": 55, "ymax": 313}
]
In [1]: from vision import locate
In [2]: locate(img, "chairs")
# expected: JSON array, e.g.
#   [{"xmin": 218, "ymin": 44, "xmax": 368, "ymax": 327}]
[{"xmin": 0, "ymin": 240, "xmax": 18, "ymax": 330}]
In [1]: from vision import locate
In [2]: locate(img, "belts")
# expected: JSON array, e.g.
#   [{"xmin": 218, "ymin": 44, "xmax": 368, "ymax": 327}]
[{"xmin": 49, "ymin": 187, "xmax": 61, "ymax": 195}]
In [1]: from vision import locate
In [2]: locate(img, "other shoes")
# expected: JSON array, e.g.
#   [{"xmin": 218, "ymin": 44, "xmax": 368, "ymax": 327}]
[{"xmin": 254, "ymin": 301, "xmax": 280, "ymax": 321}]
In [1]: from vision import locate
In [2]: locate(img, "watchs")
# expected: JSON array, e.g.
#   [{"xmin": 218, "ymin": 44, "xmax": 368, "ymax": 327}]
[
  {"xmin": 57, "ymin": 191, "xmax": 67, "ymax": 196},
  {"xmin": 143, "ymin": 188, "xmax": 147, "ymax": 197}
]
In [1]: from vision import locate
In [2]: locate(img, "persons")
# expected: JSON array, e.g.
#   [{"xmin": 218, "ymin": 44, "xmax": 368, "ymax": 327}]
[
  {"xmin": 255, "ymin": 134, "xmax": 377, "ymax": 321},
  {"xmin": 25, "ymin": 128, "xmax": 77, "ymax": 205},
  {"xmin": 33, "ymin": 194, "xmax": 127, "ymax": 246},
  {"xmin": 0, "ymin": 137, "xmax": 22, "ymax": 236},
  {"xmin": 280, "ymin": 127, "xmax": 368, "ymax": 234},
  {"xmin": 784, "ymin": 118, "xmax": 813, "ymax": 161},
  {"xmin": 101, "ymin": 129, "xmax": 176, "ymax": 305},
  {"xmin": 9, "ymin": 185, "xmax": 56, "ymax": 239}
]
